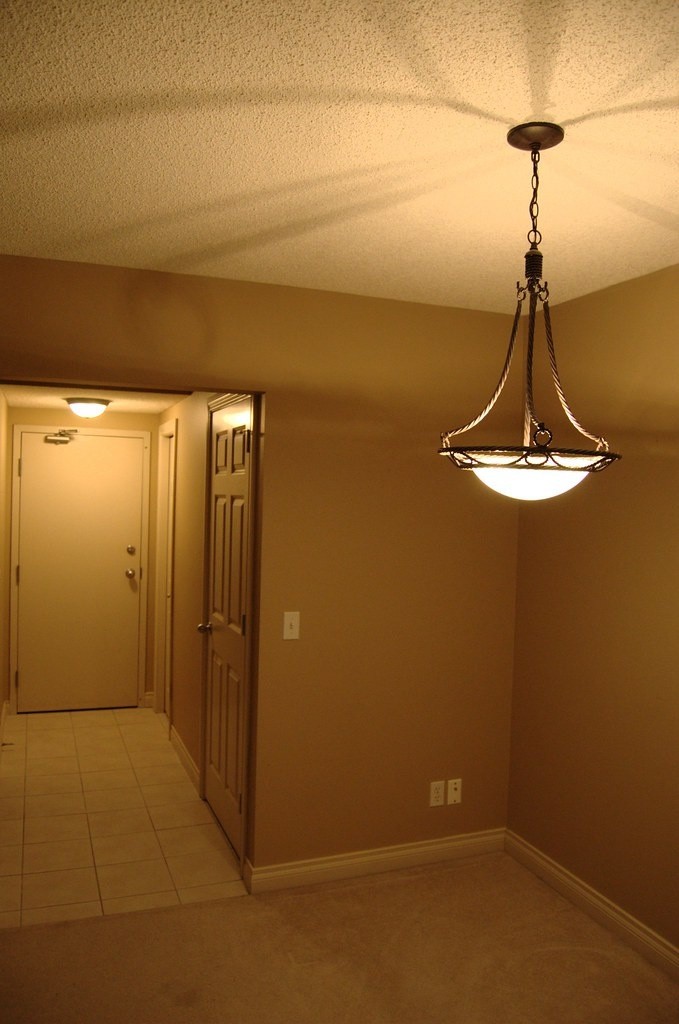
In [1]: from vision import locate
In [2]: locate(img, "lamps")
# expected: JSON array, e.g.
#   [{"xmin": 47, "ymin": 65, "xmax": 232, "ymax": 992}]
[
  {"xmin": 438, "ymin": 122, "xmax": 623, "ymax": 500},
  {"xmin": 62, "ymin": 397, "xmax": 112, "ymax": 419}
]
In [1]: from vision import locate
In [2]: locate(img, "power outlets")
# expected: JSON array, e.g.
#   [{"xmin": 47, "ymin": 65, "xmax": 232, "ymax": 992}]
[{"xmin": 430, "ymin": 781, "xmax": 445, "ymax": 807}]
